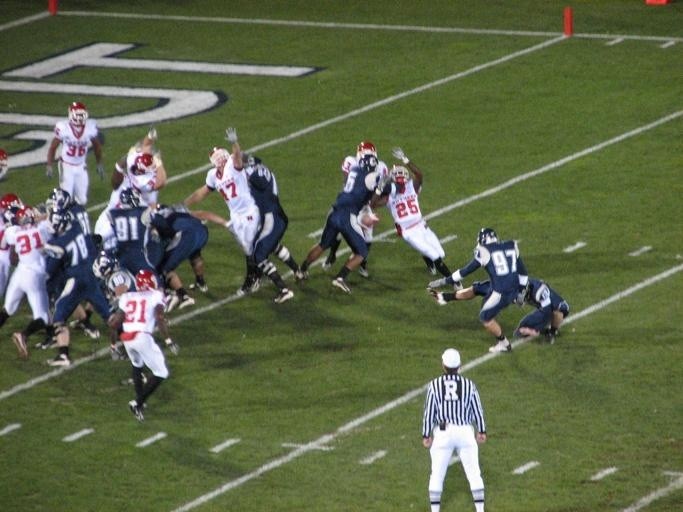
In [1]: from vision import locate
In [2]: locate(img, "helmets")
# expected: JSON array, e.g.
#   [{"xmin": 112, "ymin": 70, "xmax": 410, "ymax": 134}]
[
  {"xmin": 477, "ymin": 228, "xmax": 497, "ymax": 246},
  {"xmin": 355, "ymin": 140, "xmax": 409, "ymax": 183},
  {"xmin": 0, "ymin": 101, "xmax": 261, "ymax": 237}
]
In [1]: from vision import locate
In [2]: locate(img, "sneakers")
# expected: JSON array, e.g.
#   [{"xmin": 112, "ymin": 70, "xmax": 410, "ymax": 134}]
[{"xmin": 426, "ymin": 263, "xmax": 560, "ymax": 354}]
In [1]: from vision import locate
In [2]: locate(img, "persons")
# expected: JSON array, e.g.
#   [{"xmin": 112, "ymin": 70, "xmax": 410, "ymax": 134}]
[
  {"xmin": 109, "ymin": 268, "xmax": 178, "ymax": 420},
  {"xmin": 428, "ymin": 227, "xmax": 528, "ymax": 352},
  {"xmin": 422, "ymin": 348, "xmax": 487, "ymax": 512},
  {"xmin": 45, "ymin": 102, "xmax": 105, "ymax": 206},
  {"xmin": 178, "ymin": 127, "xmax": 303, "ymax": 303},
  {"xmin": 2, "ymin": 127, "xmax": 208, "ymax": 367},
  {"xmin": 513, "ymin": 277, "xmax": 570, "ymax": 346},
  {"xmin": 296, "ymin": 143, "xmax": 463, "ymax": 293}
]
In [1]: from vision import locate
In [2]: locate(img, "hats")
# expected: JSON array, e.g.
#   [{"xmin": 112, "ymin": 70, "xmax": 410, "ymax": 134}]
[{"xmin": 441, "ymin": 347, "xmax": 462, "ymax": 368}]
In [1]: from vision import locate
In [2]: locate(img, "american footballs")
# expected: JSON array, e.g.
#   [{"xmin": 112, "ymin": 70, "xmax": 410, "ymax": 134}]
[{"xmin": 362, "ymin": 212, "xmax": 381, "ymax": 226}]
